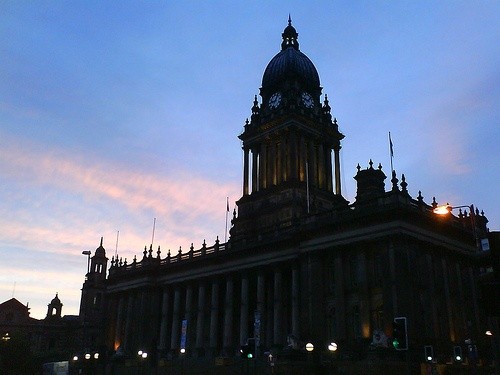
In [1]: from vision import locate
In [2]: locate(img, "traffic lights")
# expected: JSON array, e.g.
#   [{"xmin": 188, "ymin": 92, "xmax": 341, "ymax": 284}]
[
  {"xmin": 247, "ymin": 338, "xmax": 256, "ymax": 360},
  {"xmin": 453, "ymin": 346, "xmax": 462, "ymax": 362},
  {"xmin": 393, "ymin": 317, "xmax": 408, "ymax": 352},
  {"xmin": 423, "ymin": 345, "xmax": 434, "ymax": 362}
]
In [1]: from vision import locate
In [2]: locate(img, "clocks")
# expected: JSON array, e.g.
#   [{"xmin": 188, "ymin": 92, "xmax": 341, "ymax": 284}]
[
  {"xmin": 269, "ymin": 92, "xmax": 282, "ymax": 110},
  {"xmin": 302, "ymin": 92, "xmax": 315, "ymax": 109}
]
